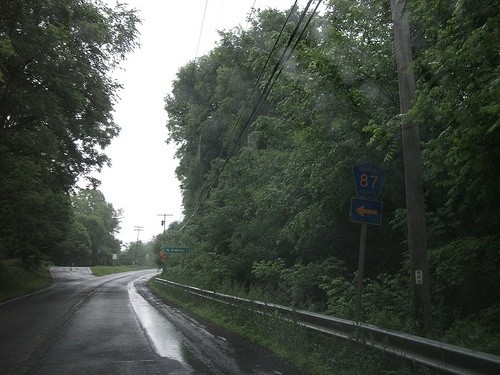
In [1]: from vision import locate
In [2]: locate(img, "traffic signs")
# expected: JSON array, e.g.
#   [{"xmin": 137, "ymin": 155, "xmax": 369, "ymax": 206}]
[{"xmin": 165, "ymin": 247, "xmax": 190, "ymax": 254}]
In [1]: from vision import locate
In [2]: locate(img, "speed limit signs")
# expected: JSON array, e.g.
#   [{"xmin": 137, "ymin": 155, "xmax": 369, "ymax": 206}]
[{"xmin": 352, "ymin": 164, "xmax": 386, "ymax": 200}]
